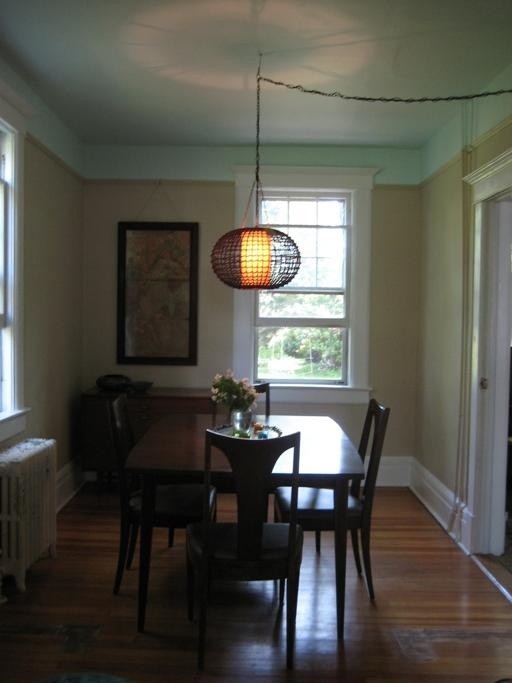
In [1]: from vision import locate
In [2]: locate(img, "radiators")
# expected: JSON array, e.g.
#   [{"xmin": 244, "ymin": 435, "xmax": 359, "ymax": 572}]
[{"xmin": 0, "ymin": 437, "xmax": 58, "ymax": 591}]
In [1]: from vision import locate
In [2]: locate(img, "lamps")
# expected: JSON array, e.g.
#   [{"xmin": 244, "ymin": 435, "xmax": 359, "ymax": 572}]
[{"xmin": 211, "ymin": 68, "xmax": 300, "ymax": 290}]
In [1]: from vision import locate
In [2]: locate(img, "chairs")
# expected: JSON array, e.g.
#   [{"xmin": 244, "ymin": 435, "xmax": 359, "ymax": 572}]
[
  {"xmin": 184, "ymin": 427, "xmax": 304, "ymax": 670},
  {"xmin": 110, "ymin": 393, "xmax": 218, "ymax": 633},
  {"xmin": 273, "ymin": 397, "xmax": 390, "ymax": 604},
  {"xmin": 210, "ymin": 384, "xmax": 274, "ymax": 416}
]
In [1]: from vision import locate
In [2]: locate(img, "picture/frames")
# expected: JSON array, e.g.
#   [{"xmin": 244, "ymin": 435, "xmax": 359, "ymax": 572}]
[{"xmin": 116, "ymin": 220, "xmax": 199, "ymax": 366}]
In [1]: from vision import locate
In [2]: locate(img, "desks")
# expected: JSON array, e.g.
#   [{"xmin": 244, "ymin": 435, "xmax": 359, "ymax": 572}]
[
  {"xmin": 80, "ymin": 388, "xmax": 216, "ymax": 495},
  {"xmin": 122, "ymin": 413, "xmax": 366, "ymax": 652}
]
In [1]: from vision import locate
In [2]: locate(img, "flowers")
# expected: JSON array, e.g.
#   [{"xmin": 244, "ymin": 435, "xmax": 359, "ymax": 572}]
[{"xmin": 210, "ymin": 369, "xmax": 258, "ymax": 410}]
